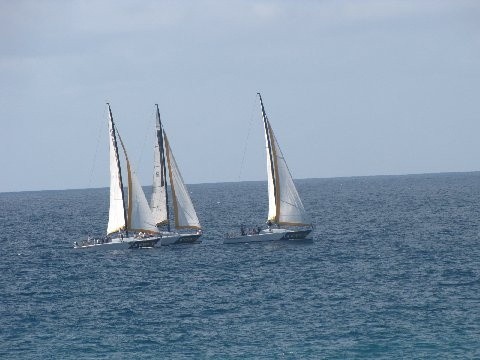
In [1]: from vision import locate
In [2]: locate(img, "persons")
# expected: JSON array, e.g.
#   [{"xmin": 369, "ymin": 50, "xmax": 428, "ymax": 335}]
[
  {"xmin": 87, "ymin": 234, "xmax": 94, "ymax": 245},
  {"xmin": 134, "ymin": 230, "xmax": 153, "ymax": 238},
  {"xmin": 241, "ymin": 223, "xmax": 245, "ymax": 235},
  {"xmin": 265, "ymin": 220, "xmax": 272, "ymax": 231}
]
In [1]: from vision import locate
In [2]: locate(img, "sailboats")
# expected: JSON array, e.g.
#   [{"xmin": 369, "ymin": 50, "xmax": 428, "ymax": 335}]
[
  {"xmin": 149, "ymin": 104, "xmax": 202, "ymax": 244},
  {"xmin": 73, "ymin": 102, "xmax": 182, "ymax": 248},
  {"xmin": 222, "ymin": 92, "xmax": 313, "ymax": 244}
]
